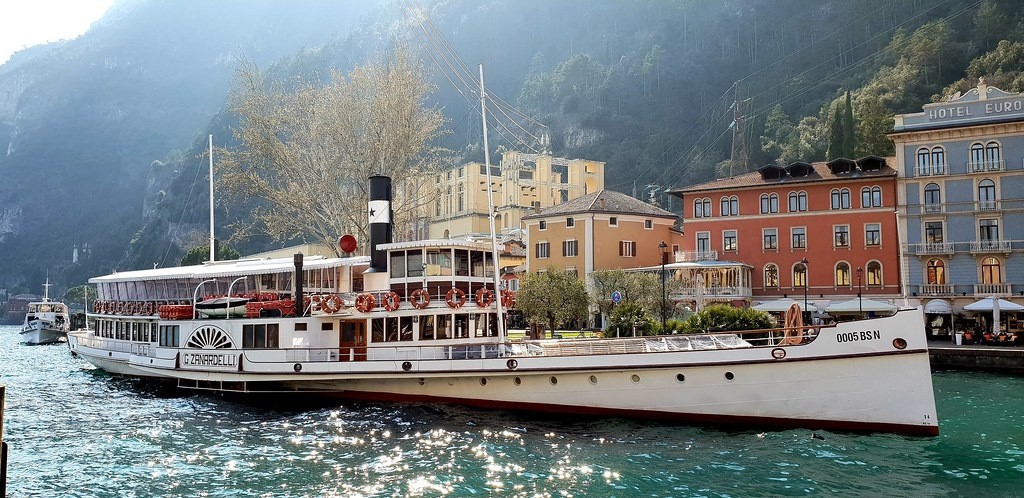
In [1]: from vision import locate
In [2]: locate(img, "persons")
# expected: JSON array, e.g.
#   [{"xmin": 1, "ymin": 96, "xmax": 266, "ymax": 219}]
[
  {"xmin": 925, "ymin": 323, "xmax": 936, "ymax": 343},
  {"xmin": 972, "ymin": 323, "xmax": 1013, "ymax": 347}
]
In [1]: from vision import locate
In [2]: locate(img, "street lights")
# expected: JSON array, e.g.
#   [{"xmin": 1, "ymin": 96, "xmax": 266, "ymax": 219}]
[
  {"xmin": 658, "ymin": 240, "xmax": 669, "ymax": 335},
  {"xmin": 801, "ymin": 257, "xmax": 809, "ymax": 331},
  {"xmin": 856, "ymin": 266, "xmax": 864, "ymax": 319}
]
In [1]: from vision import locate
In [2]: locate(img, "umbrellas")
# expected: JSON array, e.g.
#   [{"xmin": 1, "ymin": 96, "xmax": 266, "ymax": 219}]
[
  {"xmin": 964, "ymin": 298, "xmax": 1024, "ymax": 311},
  {"xmin": 749, "ymin": 298, "xmax": 897, "ymax": 312}
]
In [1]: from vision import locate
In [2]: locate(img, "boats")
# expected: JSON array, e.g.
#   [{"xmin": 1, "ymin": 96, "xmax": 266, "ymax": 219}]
[
  {"xmin": 19, "ymin": 269, "xmax": 71, "ymax": 344},
  {"xmin": 66, "ymin": 251, "xmax": 378, "ymax": 383},
  {"xmin": 124, "ymin": 237, "xmax": 947, "ymax": 441}
]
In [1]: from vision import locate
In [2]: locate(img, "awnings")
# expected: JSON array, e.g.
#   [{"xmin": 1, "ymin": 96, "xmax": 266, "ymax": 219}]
[{"xmin": 924, "ymin": 299, "xmax": 952, "ymax": 314}]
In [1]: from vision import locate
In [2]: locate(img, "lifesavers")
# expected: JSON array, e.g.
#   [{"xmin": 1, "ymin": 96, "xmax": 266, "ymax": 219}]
[
  {"xmin": 500, "ymin": 291, "xmax": 512, "ymax": 307},
  {"xmin": 355, "ymin": 294, "xmax": 376, "ymax": 313},
  {"xmin": 93, "ymin": 300, "xmax": 199, "ymax": 318},
  {"xmin": 383, "ymin": 292, "xmax": 400, "ymax": 311},
  {"xmin": 476, "ymin": 288, "xmax": 492, "ymax": 307},
  {"xmin": 446, "ymin": 288, "xmax": 465, "ymax": 309},
  {"xmin": 410, "ymin": 289, "xmax": 430, "ymax": 309},
  {"xmin": 203, "ymin": 292, "xmax": 343, "ymax": 317}
]
